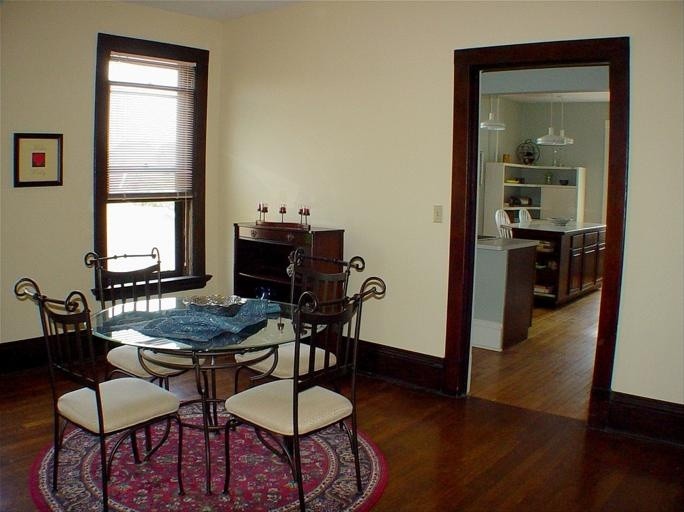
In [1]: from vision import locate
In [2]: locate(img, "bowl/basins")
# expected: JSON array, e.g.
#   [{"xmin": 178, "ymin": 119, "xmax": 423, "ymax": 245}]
[
  {"xmin": 547, "ymin": 215, "xmax": 570, "ymax": 227},
  {"xmin": 559, "ymin": 179, "xmax": 569, "ymax": 185}
]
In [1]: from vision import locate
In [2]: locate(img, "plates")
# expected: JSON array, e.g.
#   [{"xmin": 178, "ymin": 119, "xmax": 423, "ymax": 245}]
[{"xmin": 182, "ymin": 295, "xmax": 248, "ymax": 316}]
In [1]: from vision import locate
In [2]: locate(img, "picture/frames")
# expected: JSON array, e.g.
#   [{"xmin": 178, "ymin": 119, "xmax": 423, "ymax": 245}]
[{"xmin": 13, "ymin": 132, "xmax": 64, "ymax": 187}]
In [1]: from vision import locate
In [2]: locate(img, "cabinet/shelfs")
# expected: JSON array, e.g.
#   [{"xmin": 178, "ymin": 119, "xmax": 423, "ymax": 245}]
[
  {"xmin": 583, "ymin": 231, "xmax": 599, "ymax": 293},
  {"xmin": 568, "ymin": 232, "xmax": 583, "ymax": 295},
  {"xmin": 504, "ymin": 165, "xmax": 575, "ymax": 187},
  {"xmin": 234, "ymin": 222, "xmax": 346, "ymax": 328},
  {"xmin": 504, "ymin": 184, "xmax": 540, "ymax": 218},
  {"xmin": 598, "ymin": 229, "xmax": 606, "ymax": 283}
]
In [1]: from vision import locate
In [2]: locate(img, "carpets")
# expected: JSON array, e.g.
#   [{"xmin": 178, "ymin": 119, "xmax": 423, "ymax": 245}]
[{"xmin": 26, "ymin": 396, "xmax": 387, "ymax": 511}]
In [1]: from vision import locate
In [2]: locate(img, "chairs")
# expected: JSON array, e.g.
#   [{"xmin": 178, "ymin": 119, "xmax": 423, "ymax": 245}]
[
  {"xmin": 495, "ymin": 211, "xmax": 512, "ymax": 239},
  {"xmin": 13, "ymin": 278, "xmax": 186, "ymax": 512},
  {"xmin": 232, "ymin": 247, "xmax": 365, "ymax": 431},
  {"xmin": 223, "ymin": 277, "xmax": 386, "ymax": 512},
  {"xmin": 519, "ymin": 209, "xmax": 531, "ymax": 222},
  {"xmin": 84, "ymin": 248, "xmax": 169, "ymax": 392}
]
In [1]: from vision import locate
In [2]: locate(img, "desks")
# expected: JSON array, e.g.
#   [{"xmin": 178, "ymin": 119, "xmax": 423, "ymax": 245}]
[{"xmin": 87, "ymin": 296, "xmax": 327, "ymax": 494}]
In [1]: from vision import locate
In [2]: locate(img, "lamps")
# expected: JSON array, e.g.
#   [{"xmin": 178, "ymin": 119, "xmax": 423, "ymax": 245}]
[
  {"xmin": 558, "ymin": 98, "xmax": 574, "ymax": 145},
  {"xmin": 537, "ymin": 98, "xmax": 565, "ymax": 146},
  {"xmin": 480, "ymin": 95, "xmax": 506, "ymax": 131}
]
